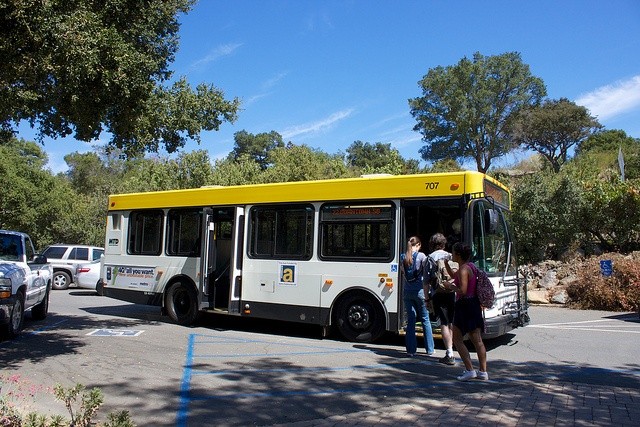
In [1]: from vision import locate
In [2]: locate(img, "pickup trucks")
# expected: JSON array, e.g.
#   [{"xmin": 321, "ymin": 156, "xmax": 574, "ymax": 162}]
[{"xmin": 1, "ymin": 230, "xmax": 53, "ymax": 336}]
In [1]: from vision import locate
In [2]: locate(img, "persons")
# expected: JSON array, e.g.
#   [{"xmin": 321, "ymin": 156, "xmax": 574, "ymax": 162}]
[
  {"xmin": 442, "ymin": 242, "xmax": 489, "ymax": 382},
  {"xmin": 399, "ymin": 236, "xmax": 437, "ymax": 357},
  {"xmin": 423, "ymin": 233, "xmax": 456, "ymax": 365}
]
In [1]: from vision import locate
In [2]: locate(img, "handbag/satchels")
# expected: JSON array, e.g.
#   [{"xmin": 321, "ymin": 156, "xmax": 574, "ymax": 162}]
[{"xmin": 477, "ymin": 269, "xmax": 495, "ymax": 309}]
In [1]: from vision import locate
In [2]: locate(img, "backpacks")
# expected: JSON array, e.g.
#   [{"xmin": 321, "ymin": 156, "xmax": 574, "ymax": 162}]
[{"xmin": 428, "ymin": 254, "xmax": 460, "ymax": 293}]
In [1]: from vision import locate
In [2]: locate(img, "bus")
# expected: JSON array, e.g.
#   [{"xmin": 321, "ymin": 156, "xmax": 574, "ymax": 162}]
[{"xmin": 101, "ymin": 171, "xmax": 530, "ymax": 344}]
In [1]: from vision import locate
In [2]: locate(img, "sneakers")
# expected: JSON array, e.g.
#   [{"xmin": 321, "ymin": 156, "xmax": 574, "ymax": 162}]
[
  {"xmin": 427, "ymin": 348, "xmax": 436, "ymax": 356},
  {"xmin": 445, "ymin": 354, "xmax": 456, "ymax": 364},
  {"xmin": 464, "ymin": 370, "xmax": 489, "ymax": 380},
  {"xmin": 457, "ymin": 370, "xmax": 477, "ymax": 381},
  {"xmin": 439, "ymin": 352, "xmax": 448, "ymax": 362},
  {"xmin": 407, "ymin": 352, "xmax": 418, "ymax": 357}
]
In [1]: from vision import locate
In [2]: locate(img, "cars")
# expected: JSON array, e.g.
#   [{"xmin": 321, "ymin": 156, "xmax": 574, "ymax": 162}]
[{"xmin": 77, "ymin": 258, "xmax": 102, "ymax": 294}]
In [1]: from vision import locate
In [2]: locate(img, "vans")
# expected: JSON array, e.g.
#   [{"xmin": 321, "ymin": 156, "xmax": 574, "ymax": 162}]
[{"xmin": 41, "ymin": 244, "xmax": 104, "ymax": 290}]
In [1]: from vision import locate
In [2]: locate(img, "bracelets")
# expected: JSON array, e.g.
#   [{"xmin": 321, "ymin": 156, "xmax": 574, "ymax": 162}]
[{"xmin": 451, "ymin": 285, "xmax": 454, "ymax": 289}]
